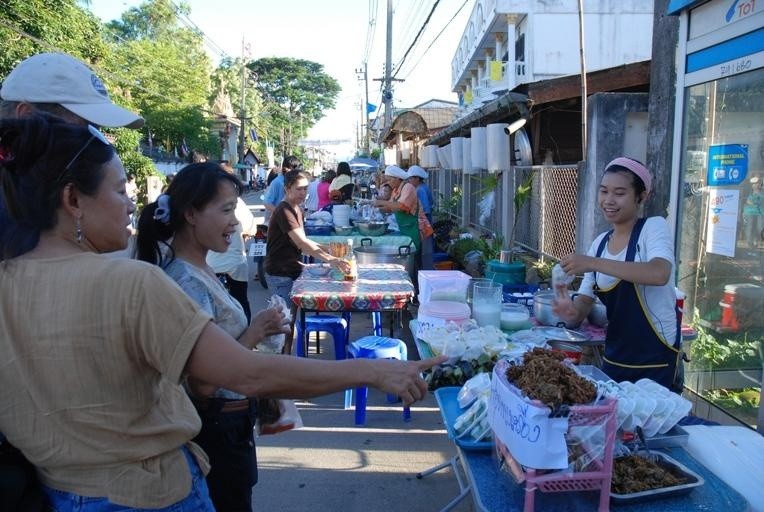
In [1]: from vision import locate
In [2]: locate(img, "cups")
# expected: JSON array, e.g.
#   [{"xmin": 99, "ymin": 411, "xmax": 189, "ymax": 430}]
[
  {"xmin": 544, "ymin": 338, "xmax": 584, "ymax": 365},
  {"xmin": 331, "ymin": 203, "xmax": 350, "ymax": 225},
  {"xmin": 473, "ymin": 280, "xmax": 504, "ymax": 330},
  {"xmin": 500, "ymin": 249, "xmax": 513, "ymax": 264}
]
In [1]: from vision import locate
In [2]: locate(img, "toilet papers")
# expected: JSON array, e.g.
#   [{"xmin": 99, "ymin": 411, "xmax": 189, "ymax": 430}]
[{"xmin": 420, "ymin": 122, "xmax": 509, "ymax": 171}]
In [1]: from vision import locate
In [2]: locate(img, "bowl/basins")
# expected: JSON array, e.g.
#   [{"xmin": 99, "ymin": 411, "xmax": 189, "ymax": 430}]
[
  {"xmin": 352, "ymin": 219, "xmax": 390, "ymax": 237},
  {"xmin": 332, "ymin": 225, "xmax": 353, "ymax": 236},
  {"xmin": 309, "ymin": 266, "xmax": 331, "ymax": 275},
  {"xmin": 499, "ymin": 300, "xmax": 531, "ymax": 330}
]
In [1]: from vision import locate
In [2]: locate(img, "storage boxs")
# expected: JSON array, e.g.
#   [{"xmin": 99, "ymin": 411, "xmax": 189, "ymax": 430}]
[
  {"xmin": 719, "ymin": 283, "xmax": 764, "ymax": 330},
  {"xmin": 673, "ymin": 286, "xmax": 687, "ymax": 332},
  {"xmin": 417, "ymin": 268, "xmax": 472, "ymax": 303},
  {"xmin": 678, "ymin": 423, "xmax": 764, "ymax": 510}
]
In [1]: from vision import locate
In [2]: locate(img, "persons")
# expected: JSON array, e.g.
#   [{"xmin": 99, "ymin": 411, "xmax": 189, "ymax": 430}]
[
  {"xmin": 547, "ymin": 151, "xmax": 691, "ymax": 400},
  {"xmin": 0, "ymin": 116, "xmax": 455, "ymax": 512},
  {"xmin": 740, "ymin": 174, "xmax": 763, "ymax": 251},
  {"xmin": 119, "ymin": 151, "xmax": 435, "ymax": 357},
  {"xmin": 124, "ymin": 158, "xmax": 293, "ymax": 512},
  {"xmin": 1, "ymin": 54, "xmax": 147, "ymax": 511}
]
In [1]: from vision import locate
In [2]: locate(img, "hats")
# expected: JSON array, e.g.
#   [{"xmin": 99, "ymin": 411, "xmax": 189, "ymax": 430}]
[
  {"xmin": 1, "ymin": 52, "xmax": 144, "ymax": 131},
  {"xmin": 406, "ymin": 165, "xmax": 429, "ymax": 180},
  {"xmin": 385, "ymin": 165, "xmax": 408, "ymax": 180}
]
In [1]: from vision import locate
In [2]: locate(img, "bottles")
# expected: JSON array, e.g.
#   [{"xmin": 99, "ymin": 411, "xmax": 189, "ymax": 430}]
[{"xmin": 342, "ymin": 238, "xmax": 358, "ymax": 283}]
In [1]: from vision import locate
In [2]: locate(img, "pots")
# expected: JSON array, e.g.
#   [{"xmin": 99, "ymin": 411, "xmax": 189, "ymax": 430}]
[
  {"xmin": 350, "ymin": 236, "xmax": 417, "ymax": 278},
  {"xmin": 533, "ymin": 287, "xmax": 583, "ymax": 330},
  {"xmin": 585, "ymin": 301, "xmax": 609, "ymax": 327}
]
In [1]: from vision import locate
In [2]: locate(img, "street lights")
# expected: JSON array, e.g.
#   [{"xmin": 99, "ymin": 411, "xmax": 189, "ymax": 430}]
[{"xmin": 238, "ymin": 34, "xmax": 251, "ymax": 164}]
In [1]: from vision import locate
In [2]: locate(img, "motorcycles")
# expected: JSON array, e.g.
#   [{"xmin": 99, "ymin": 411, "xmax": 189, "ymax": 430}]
[
  {"xmin": 249, "ymin": 192, "xmax": 311, "ymax": 290},
  {"xmin": 253, "ymin": 176, "xmax": 267, "ymax": 192}
]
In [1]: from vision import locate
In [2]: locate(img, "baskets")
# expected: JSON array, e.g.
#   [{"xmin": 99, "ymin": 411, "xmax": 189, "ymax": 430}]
[
  {"xmin": 502, "ymin": 284, "xmax": 543, "ymax": 306},
  {"xmin": 304, "ymin": 218, "xmax": 331, "ymax": 236}
]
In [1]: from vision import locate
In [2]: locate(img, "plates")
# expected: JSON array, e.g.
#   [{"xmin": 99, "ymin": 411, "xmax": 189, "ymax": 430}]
[
  {"xmin": 419, "ymin": 300, "xmax": 472, "ymax": 325},
  {"xmin": 530, "ymin": 325, "xmax": 590, "ymax": 344}
]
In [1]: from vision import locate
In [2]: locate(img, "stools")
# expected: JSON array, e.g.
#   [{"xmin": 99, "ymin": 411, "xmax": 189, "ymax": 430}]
[
  {"xmin": 344, "ymin": 337, "xmax": 411, "ymax": 425},
  {"xmin": 342, "ymin": 311, "xmax": 382, "ymax": 337},
  {"xmin": 295, "ymin": 314, "xmax": 347, "ymax": 360}
]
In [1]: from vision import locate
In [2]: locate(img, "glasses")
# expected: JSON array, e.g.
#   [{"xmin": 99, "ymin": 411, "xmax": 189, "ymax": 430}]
[{"xmin": 60, "ymin": 125, "xmax": 109, "ymax": 183}]
